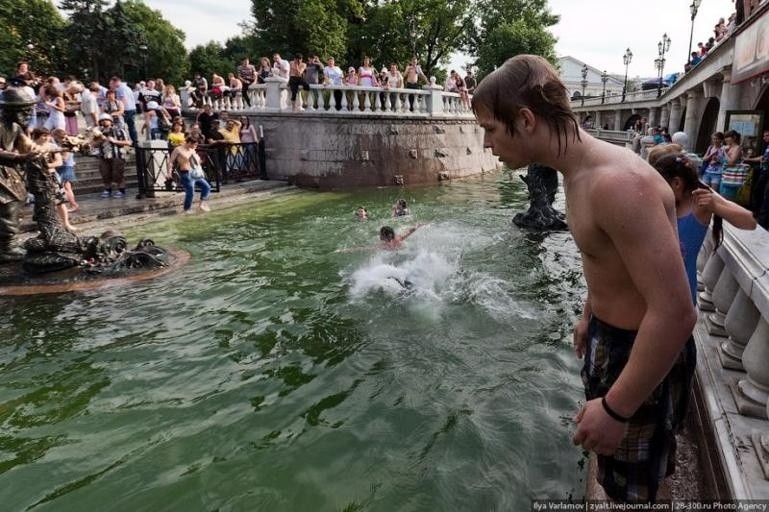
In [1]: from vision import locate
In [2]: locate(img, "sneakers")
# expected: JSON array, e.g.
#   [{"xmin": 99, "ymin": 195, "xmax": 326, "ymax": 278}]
[
  {"xmin": 112, "ymin": 188, "xmax": 130, "ymax": 199},
  {"xmin": 99, "ymin": 188, "xmax": 112, "ymax": 199}
]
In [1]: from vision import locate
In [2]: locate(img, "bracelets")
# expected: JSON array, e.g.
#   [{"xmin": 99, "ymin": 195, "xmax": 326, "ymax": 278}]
[{"xmin": 598, "ymin": 394, "xmax": 633, "ymax": 425}]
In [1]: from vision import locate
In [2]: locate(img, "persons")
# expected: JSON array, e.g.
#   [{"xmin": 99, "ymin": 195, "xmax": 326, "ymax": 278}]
[
  {"xmin": 684, "ymin": 11, "xmax": 736, "ymax": 72},
  {"xmin": 647, "ymin": 143, "xmax": 687, "ymax": 166},
  {"xmin": 632, "ymin": 116, "xmax": 673, "ymax": 146},
  {"xmin": 643, "ymin": 141, "xmax": 759, "ymax": 437},
  {"xmin": 355, "ymin": 206, "xmax": 368, "ymax": 221},
  {"xmin": 333, "ymin": 219, "xmax": 430, "ymax": 255},
  {"xmin": 257, "ymin": 53, "xmax": 478, "ymax": 115},
  {"xmin": 703, "ymin": 129, "xmax": 769, "ymax": 232},
  {"xmin": 391, "ymin": 200, "xmax": 411, "ymax": 217},
  {"xmin": 0, "ymin": 86, "xmax": 57, "ymax": 257},
  {"xmin": 467, "ymin": 53, "xmax": 700, "ymax": 510},
  {"xmin": 1, "ymin": 56, "xmax": 262, "ymax": 233},
  {"xmin": 581, "ymin": 115, "xmax": 593, "ymax": 129}
]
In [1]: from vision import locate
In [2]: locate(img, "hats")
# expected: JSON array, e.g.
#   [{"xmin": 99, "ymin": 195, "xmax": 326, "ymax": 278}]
[{"xmin": 97, "ymin": 111, "xmax": 116, "ymax": 123}]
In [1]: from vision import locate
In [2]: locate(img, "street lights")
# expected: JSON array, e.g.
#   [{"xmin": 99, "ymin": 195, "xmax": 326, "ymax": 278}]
[
  {"xmin": 600, "ymin": 71, "xmax": 609, "ymax": 101},
  {"xmin": 580, "ymin": 63, "xmax": 590, "ymax": 103},
  {"xmin": 687, "ymin": 0, "xmax": 702, "ymax": 62},
  {"xmin": 137, "ymin": 44, "xmax": 149, "ymax": 80},
  {"xmin": 653, "ymin": 33, "xmax": 673, "ymax": 98},
  {"xmin": 655, "ymin": 55, "xmax": 666, "ymax": 96},
  {"xmin": 622, "ymin": 48, "xmax": 634, "ymax": 102}
]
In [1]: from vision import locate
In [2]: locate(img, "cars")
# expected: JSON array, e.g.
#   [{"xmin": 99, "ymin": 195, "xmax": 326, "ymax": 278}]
[{"xmin": 642, "ymin": 79, "xmax": 668, "ymax": 96}]
